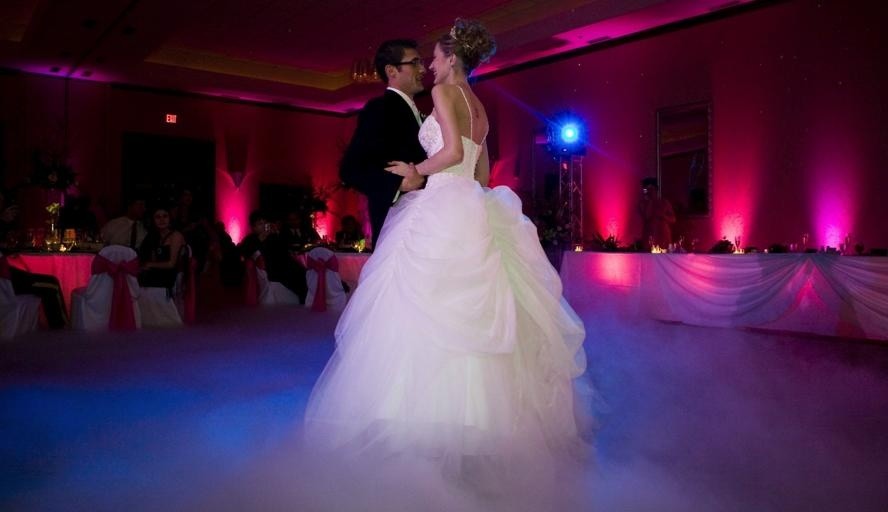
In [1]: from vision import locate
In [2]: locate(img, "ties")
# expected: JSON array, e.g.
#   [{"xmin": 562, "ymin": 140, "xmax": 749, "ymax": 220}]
[{"xmin": 130, "ymin": 222, "xmax": 137, "ymax": 250}]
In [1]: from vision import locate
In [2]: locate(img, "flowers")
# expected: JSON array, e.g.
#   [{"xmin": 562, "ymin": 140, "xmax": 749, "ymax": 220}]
[{"xmin": 45, "ymin": 201, "xmax": 61, "ymax": 219}]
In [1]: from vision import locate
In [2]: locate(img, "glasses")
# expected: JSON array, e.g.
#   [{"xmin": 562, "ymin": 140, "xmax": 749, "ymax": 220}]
[{"xmin": 396, "ymin": 58, "xmax": 425, "ymax": 66}]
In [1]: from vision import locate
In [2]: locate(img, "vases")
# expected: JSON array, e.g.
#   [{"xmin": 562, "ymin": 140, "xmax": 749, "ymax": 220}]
[{"xmin": 43, "ymin": 216, "xmax": 64, "ymax": 251}]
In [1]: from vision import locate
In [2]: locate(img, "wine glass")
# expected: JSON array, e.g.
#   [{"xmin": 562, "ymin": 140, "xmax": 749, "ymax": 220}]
[
  {"xmin": 644, "ymin": 233, "xmax": 865, "ymax": 257},
  {"xmin": 61, "ymin": 236, "xmax": 75, "ymax": 254}
]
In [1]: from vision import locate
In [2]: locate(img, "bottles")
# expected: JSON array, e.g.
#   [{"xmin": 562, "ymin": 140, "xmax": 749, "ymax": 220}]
[{"xmin": 45, "ymin": 217, "xmax": 61, "ymax": 251}]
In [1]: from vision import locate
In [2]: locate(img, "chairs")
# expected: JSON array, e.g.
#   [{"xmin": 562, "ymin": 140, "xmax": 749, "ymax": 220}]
[
  {"xmin": 0, "ymin": 252, "xmax": 49, "ymax": 342},
  {"xmin": 139, "ymin": 242, "xmax": 192, "ymax": 326},
  {"xmin": 243, "ymin": 252, "xmax": 301, "ymax": 304},
  {"xmin": 299, "ymin": 248, "xmax": 350, "ymax": 312},
  {"xmin": 77, "ymin": 246, "xmax": 144, "ymax": 332}
]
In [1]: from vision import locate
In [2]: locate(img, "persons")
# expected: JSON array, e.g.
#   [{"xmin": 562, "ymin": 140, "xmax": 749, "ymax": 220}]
[
  {"xmin": 638, "ymin": 177, "xmax": 676, "ymax": 250},
  {"xmin": 0, "ymin": 188, "xmax": 365, "ymax": 331},
  {"xmin": 307, "ymin": 16, "xmax": 591, "ymax": 509},
  {"xmin": 337, "ymin": 38, "xmax": 428, "ymax": 255}
]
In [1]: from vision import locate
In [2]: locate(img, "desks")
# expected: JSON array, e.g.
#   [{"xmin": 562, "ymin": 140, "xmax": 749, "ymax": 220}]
[{"xmin": 560, "ymin": 249, "xmax": 887, "ymax": 347}]
